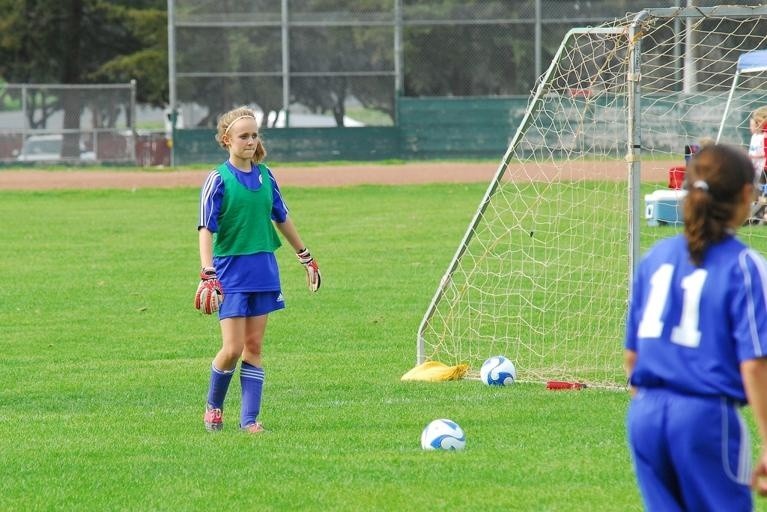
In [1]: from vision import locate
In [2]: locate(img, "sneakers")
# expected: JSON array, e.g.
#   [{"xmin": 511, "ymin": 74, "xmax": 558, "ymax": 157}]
[
  {"xmin": 241, "ymin": 423, "xmax": 265, "ymax": 434},
  {"xmin": 204, "ymin": 407, "xmax": 223, "ymax": 433}
]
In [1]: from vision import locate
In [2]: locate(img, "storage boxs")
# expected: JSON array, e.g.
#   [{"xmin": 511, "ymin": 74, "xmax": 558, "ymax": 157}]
[{"xmin": 643, "ymin": 190, "xmax": 689, "ymax": 228}]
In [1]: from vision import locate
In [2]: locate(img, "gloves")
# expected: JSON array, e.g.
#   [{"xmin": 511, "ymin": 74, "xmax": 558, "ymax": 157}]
[
  {"xmin": 194, "ymin": 268, "xmax": 224, "ymax": 315},
  {"xmin": 296, "ymin": 248, "xmax": 321, "ymax": 293}
]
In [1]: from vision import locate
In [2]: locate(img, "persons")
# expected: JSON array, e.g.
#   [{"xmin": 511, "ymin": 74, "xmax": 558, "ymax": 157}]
[
  {"xmin": 191, "ymin": 105, "xmax": 320, "ymax": 435},
  {"xmin": 750, "ymin": 106, "xmax": 766, "ymax": 206},
  {"xmin": 620, "ymin": 140, "xmax": 767, "ymax": 511},
  {"xmin": 742, "ymin": 118, "xmax": 765, "ymax": 187}
]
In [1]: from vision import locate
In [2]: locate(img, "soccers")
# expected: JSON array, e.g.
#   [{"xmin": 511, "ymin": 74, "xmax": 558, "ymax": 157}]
[
  {"xmin": 421, "ymin": 418, "xmax": 466, "ymax": 451},
  {"xmin": 480, "ymin": 356, "xmax": 517, "ymax": 385}
]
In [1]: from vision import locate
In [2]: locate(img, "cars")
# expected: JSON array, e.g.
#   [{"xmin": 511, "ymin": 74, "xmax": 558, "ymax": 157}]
[{"xmin": 11, "ymin": 135, "xmax": 97, "ymax": 163}]
